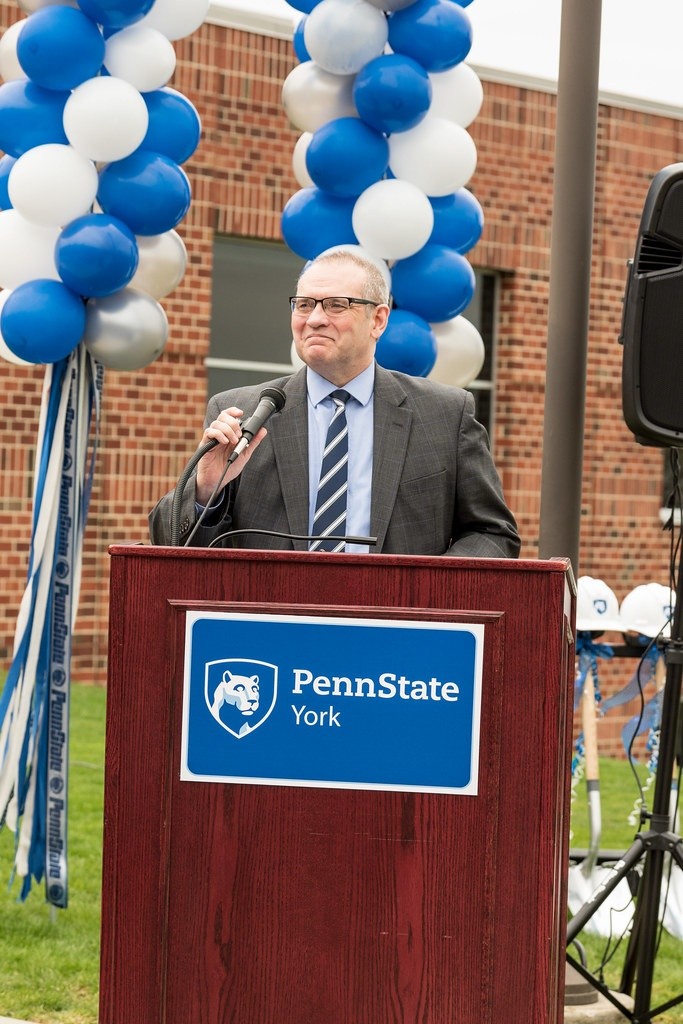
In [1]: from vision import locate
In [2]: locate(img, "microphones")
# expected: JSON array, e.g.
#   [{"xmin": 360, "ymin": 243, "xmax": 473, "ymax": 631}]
[
  {"xmin": 229, "ymin": 386, "xmax": 287, "ymax": 463},
  {"xmin": 208, "ymin": 529, "xmax": 377, "ymax": 547}
]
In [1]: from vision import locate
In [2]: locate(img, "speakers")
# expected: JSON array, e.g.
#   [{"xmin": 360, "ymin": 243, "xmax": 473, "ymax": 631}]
[{"xmin": 616, "ymin": 163, "xmax": 683, "ymax": 451}]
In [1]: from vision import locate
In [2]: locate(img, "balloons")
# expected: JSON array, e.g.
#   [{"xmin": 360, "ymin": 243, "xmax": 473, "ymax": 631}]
[{"xmin": 2, "ymin": 0, "xmax": 487, "ymax": 390}]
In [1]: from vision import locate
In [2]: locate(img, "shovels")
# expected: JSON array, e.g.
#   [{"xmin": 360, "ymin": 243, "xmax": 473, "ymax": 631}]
[
  {"xmin": 651, "ymin": 649, "xmax": 683, "ymax": 940},
  {"xmin": 567, "ymin": 649, "xmax": 635, "ymax": 939}
]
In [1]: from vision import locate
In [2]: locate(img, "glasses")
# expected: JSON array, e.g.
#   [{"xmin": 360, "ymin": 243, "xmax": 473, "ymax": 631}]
[{"xmin": 289, "ymin": 294, "xmax": 381, "ymax": 318}]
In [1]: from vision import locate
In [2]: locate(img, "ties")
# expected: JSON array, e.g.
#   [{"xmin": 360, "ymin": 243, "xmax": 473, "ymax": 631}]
[{"xmin": 310, "ymin": 389, "xmax": 353, "ymax": 553}]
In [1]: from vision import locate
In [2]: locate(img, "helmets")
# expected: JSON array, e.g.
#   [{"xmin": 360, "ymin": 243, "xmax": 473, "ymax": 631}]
[
  {"xmin": 572, "ymin": 577, "xmax": 628, "ymax": 632},
  {"xmin": 620, "ymin": 583, "xmax": 677, "ymax": 638}
]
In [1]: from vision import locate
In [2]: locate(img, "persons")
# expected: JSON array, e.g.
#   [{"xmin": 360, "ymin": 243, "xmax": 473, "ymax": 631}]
[{"xmin": 148, "ymin": 252, "xmax": 521, "ymax": 559}]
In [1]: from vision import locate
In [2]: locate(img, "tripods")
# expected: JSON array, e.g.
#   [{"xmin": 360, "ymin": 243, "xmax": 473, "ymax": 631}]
[{"xmin": 567, "ymin": 537, "xmax": 683, "ymax": 1024}]
